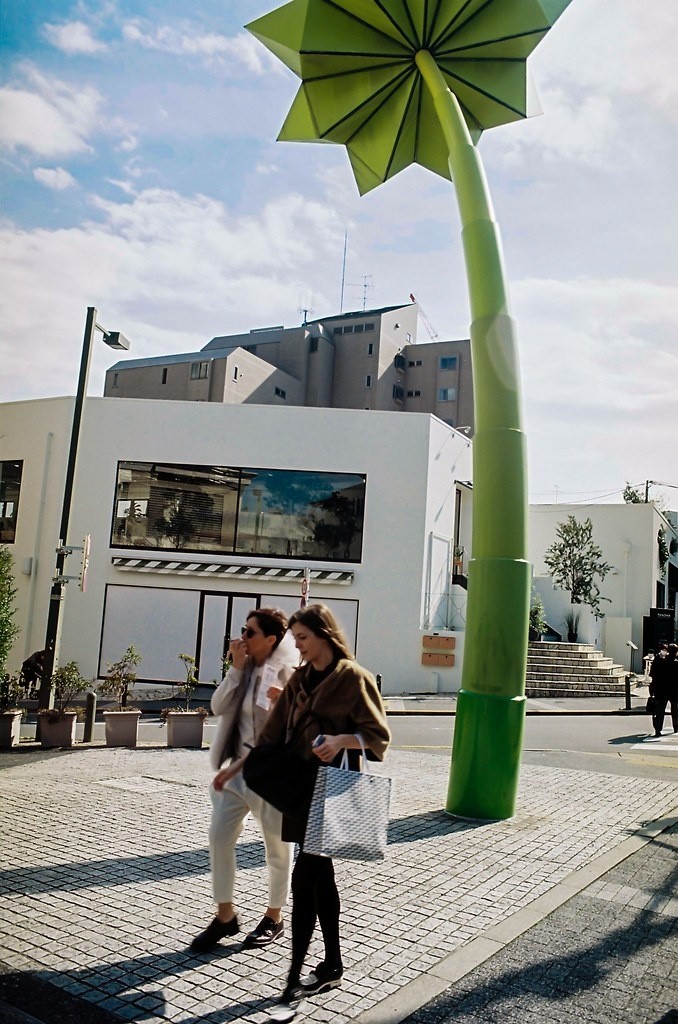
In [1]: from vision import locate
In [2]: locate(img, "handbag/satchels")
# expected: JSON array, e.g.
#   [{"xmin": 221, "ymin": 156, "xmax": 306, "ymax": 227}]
[
  {"xmin": 646, "ymin": 692, "xmax": 655, "ymax": 712},
  {"xmin": 303, "ymin": 733, "xmax": 392, "ymax": 862}
]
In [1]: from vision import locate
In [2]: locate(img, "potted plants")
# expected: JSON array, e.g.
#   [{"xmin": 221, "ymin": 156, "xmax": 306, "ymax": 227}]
[
  {"xmin": 36, "ymin": 661, "xmax": 93, "ymax": 747},
  {"xmin": 560, "ymin": 609, "xmax": 580, "ymax": 643},
  {"xmin": 0, "ymin": 672, "xmax": 27, "ymax": 750},
  {"xmin": 454, "ymin": 546, "xmax": 463, "ymax": 563},
  {"xmin": 528, "ymin": 595, "xmax": 548, "ymax": 640},
  {"xmin": 99, "ymin": 643, "xmax": 144, "ymax": 748},
  {"xmin": 159, "ymin": 653, "xmax": 210, "ymax": 749}
]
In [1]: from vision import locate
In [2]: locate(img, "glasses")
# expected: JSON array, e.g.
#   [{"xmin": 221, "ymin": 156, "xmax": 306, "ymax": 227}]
[{"xmin": 240, "ymin": 626, "xmax": 264, "ymax": 639}]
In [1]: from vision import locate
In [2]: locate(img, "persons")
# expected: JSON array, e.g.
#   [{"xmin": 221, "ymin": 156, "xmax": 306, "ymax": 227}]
[
  {"xmin": 191, "ymin": 609, "xmax": 305, "ymax": 951},
  {"xmin": 21, "ymin": 650, "xmax": 48, "ymax": 700},
  {"xmin": 212, "ymin": 602, "xmax": 393, "ymax": 1002},
  {"xmin": 648, "ymin": 643, "xmax": 678, "ymax": 736}
]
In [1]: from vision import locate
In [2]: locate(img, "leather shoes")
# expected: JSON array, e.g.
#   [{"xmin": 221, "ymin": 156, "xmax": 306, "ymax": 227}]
[
  {"xmin": 244, "ymin": 915, "xmax": 285, "ymax": 946},
  {"xmin": 189, "ymin": 912, "xmax": 239, "ymax": 954}
]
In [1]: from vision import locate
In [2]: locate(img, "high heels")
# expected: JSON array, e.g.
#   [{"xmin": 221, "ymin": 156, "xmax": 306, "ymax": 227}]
[
  {"xmin": 269, "ymin": 988, "xmax": 305, "ymax": 1020},
  {"xmin": 299, "ymin": 963, "xmax": 343, "ymax": 994}
]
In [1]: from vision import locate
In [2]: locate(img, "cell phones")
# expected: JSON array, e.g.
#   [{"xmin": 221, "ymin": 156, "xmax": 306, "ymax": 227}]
[{"xmin": 312, "ymin": 735, "xmax": 334, "ymax": 764}]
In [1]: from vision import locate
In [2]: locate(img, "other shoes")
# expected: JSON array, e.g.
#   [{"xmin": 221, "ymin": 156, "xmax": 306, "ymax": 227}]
[
  {"xmin": 674, "ymin": 729, "xmax": 678, "ymax": 733},
  {"xmin": 655, "ymin": 731, "xmax": 661, "ymax": 737}
]
